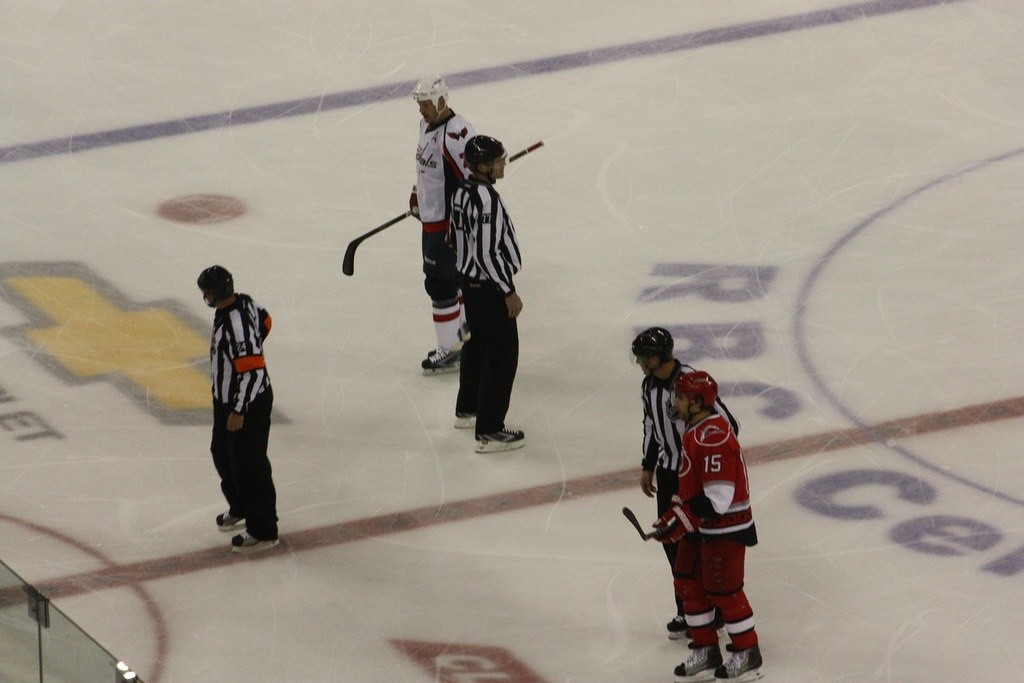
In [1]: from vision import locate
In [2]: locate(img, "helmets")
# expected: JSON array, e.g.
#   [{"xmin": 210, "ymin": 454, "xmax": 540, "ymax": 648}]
[
  {"xmin": 196, "ymin": 266, "xmax": 235, "ymax": 307},
  {"xmin": 674, "ymin": 370, "xmax": 718, "ymax": 415},
  {"xmin": 632, "ymin": 327, "xmax": 674, "ymax": 365},
  {"xmin": 463, "ymin": 135, "xmax": 504, "ymax": 174},
  {"xmin": 410, "ymin": 75, "xmax": 452, "ymax": 112}
]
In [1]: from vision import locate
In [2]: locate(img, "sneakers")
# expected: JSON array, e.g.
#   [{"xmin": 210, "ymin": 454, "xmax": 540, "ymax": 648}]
[
  {"xmin": 231, "ymin": 530, "xmax": 280, "ymax": 554},
  {"xmin": 674, "ymin": 640, "xmax": 722, "ymax": 683},
  {"xmin": 475, "ymin": 424, "xmax": 525, "ymax": 453},
  {"xmin": 666, "ymin": 616, "xmax": 688, "ymax": 640},
  {"xmin": 714, "ymin": 643, "xmax": 762, "ymax": 683},
  {"xmin": 455, "ymin": 409, "xmax": 477, "ymax": 428},
  {"xmin": 685, "ymin": 607, "xmax": 725, "ymax": 644},
  {"xmin": 421, "ymin": 322, "xmax": 469, "ymax": 373},
  {"xmin": 216, "ymin": 510, "xmax": 245, "ymax": 532}
]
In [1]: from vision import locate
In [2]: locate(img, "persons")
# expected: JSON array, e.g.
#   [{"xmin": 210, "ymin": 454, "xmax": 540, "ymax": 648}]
[
  {"xmin": 409, "ymin": 72, "xmax": 475, "ymax": 375},
  {"xmin": 197, "ymin": 264, "xmax": 280, "ymax": 554},
  {"xmin": 629, "ymin": 327, "xmax": 741, "ymax": 638},
  {"xmin": 652, "ymin": 372, "xmax": 765, "ymax": 683},
  {"xmin": 444, "ymin": 135, "xmax": 526, "ymax": 453}
]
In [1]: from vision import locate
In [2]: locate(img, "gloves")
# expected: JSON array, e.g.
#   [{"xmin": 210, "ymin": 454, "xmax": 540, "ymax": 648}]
[
  {"xmin": 652, "ymin": 504, "xmax": 703, "ymax": 543},
  {"xmin": 408, "ymin": 185, "xmax": 420, "ymax": 220}
]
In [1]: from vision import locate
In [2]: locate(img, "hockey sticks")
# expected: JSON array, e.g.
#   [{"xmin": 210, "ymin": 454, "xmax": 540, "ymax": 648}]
[
  {"xmin": 341, "ymin": 140, "xmax": 547, "ymax": 276},
  {"xmin": 621, "ymin": 504, "xmax": 671, "ymax": 542}
]
